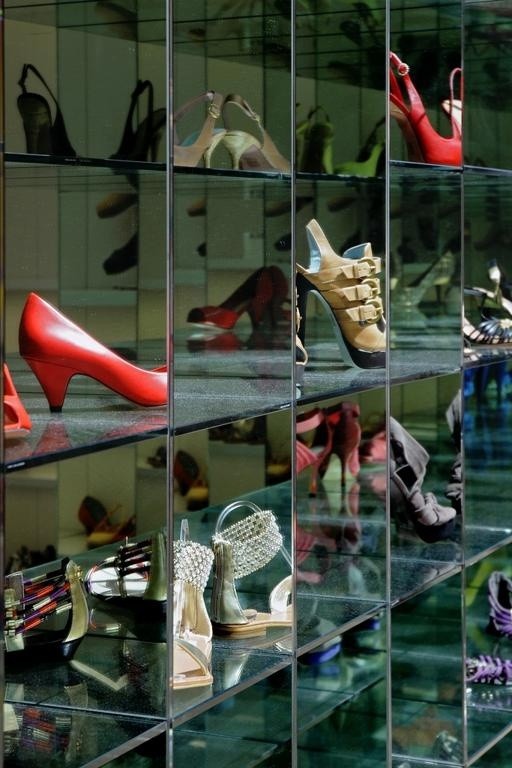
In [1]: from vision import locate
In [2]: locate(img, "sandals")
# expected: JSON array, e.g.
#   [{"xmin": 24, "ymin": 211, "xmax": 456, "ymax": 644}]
[
  {"xmin": 167, "ymin": 541, "xmax": 216, "ymax": 689},
  {"xmin": 484, "ymin": 572, "xmax": 512, "ymax": 634},
  {"xmin": 82, "ymin": 531, "xmax": 167, "ymax": 643},
  {"xmin": 465, "ymin": 655, "xmax": 512, "ymax": 687},
  {"xmin": 4, "ymin": 559, "xmax": 90, "ymax": 670},
  {"xmin": 212, "ymin": 511, "xmax": 292, "ymax": 641}
]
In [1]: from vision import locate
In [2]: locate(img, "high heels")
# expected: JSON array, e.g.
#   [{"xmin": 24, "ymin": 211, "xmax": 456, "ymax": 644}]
[
  {"xmin": 337, "ymin": 117, "xmax": 388, "ymax": 176},
  {"xmin": 295, "ymin": 305, "xmax": 312, "ymax": 385},
  {"xmin": 222, "ymin": 94, "xmax": 291, "ymax": 172},
  {"xmin": 388, "ymin": 417, "xmax": 456, "ymax": 541},
  {"xmin": 359, "ymin": 413, "xmax": 386, "ymax": 464},
  {"xmin": 462, "ymin": 260, "xmax": 512, "ymax": 343},
  {"xmin": 294, "ymin": 412, "xmax": 332, "ymax": 500},
  {"xmin": 318, "ymin": 404, "xmax": 362, "ymax": 487},
  {"xmin": 463, "ymin": 317, "xmax": 499, "ymax": 345},
  {"xmin": 18, "ymin": 64, "xmax": 76, "ymax": 156},
  {"xmin": 442, "ymin": 394, "xmax": 465, "ymax": 504},
  {"xmin": 175, "ymin": 90, "xmax": 230, "ymax": 169},
  {"xmin": 188, "ymin": 270, "xmax": 271, "ymax": 329},
  {"xmin": 19, "ymin": 292, "xmax": 168, "ymax": 412},
  {"xmin": 3, "ymin": 364, "xmax": 32, "ymax": 440},
  {"xmin": 109, "ymin": 80, "xmax": 166, "ymax": 162},
  {"xmin": 295, "ymin": 107, "xmax": 335, "ymax": 173},
  {"xmin": 296, "ymin": 219, "xmax": 389, "ymax": 367},
  {"xmin": 389, "ymin": 52, "xmax": 463, "ymax": 169},
  {"xmin": 439, "ymin": 67, "xmax": 462, "ymax": 140}
]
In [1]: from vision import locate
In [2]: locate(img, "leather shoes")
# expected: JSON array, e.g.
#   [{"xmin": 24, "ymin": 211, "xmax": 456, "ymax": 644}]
[
  {"xmin": 80, "ymin": 497, "xmax": 137, "ymax": 544},
  {"xmin": 265, "ymin": 191, "xmax": 293, "ymax": 218},
  {"xmin": 275, "ymin": 235, "xmax": 291, "ymax": 253},
  {"xmin": 186, "ymin": 201, "xmax": 207, "ymax": 218},
  {"xmin": 98, "ymin": 194, "xmax": 136, "ymax": 217},
  {"xmin": 174, "ymin": 451, "xmax": 208, "ymax": 509},
  {"xmin": 327, "ymin": 182, "xmax": 364, "ymax": 212},
  {"xmin": 102, "ymin": 231, "xmax": 138, "ymax": 277}
]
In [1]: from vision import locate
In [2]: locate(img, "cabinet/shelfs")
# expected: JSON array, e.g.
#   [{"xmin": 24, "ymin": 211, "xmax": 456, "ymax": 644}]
[{"xmin": 0, "ymin": 145, "xmax": 512, "ymax": 768}]
